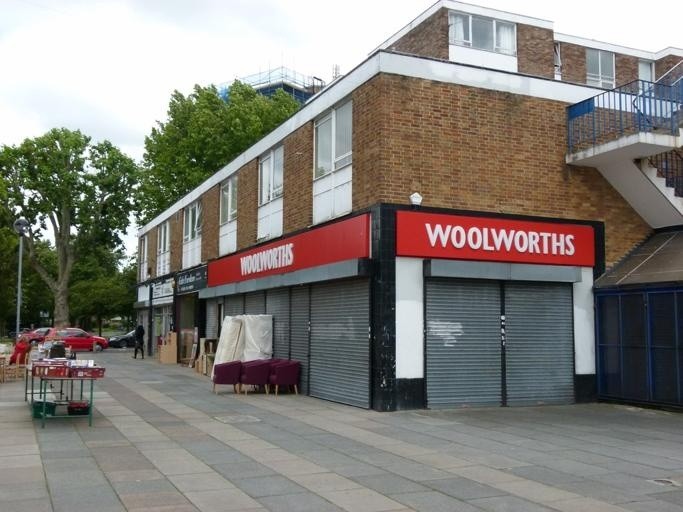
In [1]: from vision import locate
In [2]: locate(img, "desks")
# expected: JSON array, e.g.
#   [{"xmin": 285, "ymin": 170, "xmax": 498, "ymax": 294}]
[{"xmin": 23, "ymin": 366, "xmax": 93, "ymax": 426}]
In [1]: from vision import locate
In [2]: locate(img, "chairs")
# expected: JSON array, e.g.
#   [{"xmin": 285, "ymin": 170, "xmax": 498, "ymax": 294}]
[{"xmin": 210, "ymin": 356, "xmax": 299, "ymax": 395}]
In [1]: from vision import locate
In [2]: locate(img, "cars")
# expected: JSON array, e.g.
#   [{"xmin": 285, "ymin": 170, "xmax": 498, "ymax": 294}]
[{"xmin": 8, "ymin": 327, "xmax": 136, "ymax": 352}]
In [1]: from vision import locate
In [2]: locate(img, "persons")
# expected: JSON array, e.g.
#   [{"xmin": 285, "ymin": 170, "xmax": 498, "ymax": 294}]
[
  {"xmin": 134, "ymin": 325, "xmax": 145, "ymax": 359},
  {"xmin": 38, "ymin": 328, "xmax": 54, "ymax": 359},
  {"xmin": 9, "ymin": 336, "xmax": 31, "ymax": 365}
]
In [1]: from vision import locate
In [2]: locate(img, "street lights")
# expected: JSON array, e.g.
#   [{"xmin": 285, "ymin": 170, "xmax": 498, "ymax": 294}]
[{"xmin": 13, "ymin": 218, "xmax": 28, "ymax": 344}]
